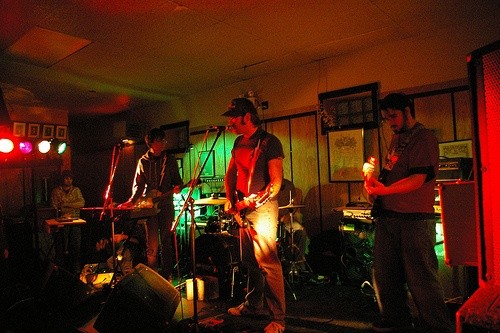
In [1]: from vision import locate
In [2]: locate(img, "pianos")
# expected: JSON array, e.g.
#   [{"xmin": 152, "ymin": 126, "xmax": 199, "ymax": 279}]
[{"xmin": 77, "ymin": 202, "xmax": 162, "ymax": 281}]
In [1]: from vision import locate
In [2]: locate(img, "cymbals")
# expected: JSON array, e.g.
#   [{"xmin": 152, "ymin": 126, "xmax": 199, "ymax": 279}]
[
  {"xmin": 189, "ymin": 196, "xmax": 229, "ymax": 206},
  {"xmin": 277, "ymin": 204, "xmax": 307, "ymax": 210}
]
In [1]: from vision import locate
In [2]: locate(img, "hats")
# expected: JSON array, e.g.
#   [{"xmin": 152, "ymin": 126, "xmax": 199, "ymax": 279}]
[
  {"xmin": 221, "ymin": 98, "xmax": 256, "ymax": 118},
  {"xmin": 62, "ymin": 170, "xmax": 73, "ymax": 176}
]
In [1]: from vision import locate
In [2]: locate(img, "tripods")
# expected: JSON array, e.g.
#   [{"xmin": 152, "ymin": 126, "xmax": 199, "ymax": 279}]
[{"xmin": 276, "ymin": 209, "xmax": 317, "ymax": 302}]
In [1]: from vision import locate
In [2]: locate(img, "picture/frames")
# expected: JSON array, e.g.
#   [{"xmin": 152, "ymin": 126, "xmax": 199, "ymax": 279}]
[
  {"xmin": 27, "ymin": 124, "xmax": 40, "ymax": 137},
  {"xmin": 326, "ymin": 128, "xmax": 366, "ymax": 183},
  {"xmin": 13, "ymin": 122, "xmax": 25, "ymax": 137},
  {"xmin": 56, "ymin": 126, "xmax": 66, "ymax": 139},
  {"xmin": 42, "ymin": 125, "xmax": 54, "ymax": 138},
  {"xmin": 319, "ymin": 82, "xmax": 380, "ymax": 135}
]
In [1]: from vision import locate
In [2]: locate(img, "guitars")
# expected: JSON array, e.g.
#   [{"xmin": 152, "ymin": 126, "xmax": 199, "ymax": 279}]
[
  {"xmin": 146, "ymin": 179, "xmax": 201, "ymax": 202},
  {"xmin": 218, "ymin": 185, "xmax": 273, "ymax": 228}
]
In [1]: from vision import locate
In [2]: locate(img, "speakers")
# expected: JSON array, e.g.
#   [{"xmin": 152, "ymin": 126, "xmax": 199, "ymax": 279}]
[
  {"xmin": 37, "ymin": 208, "xmax": 58, "ymax": 259},
  {"xmin": 41, "ymin": 265, "xmax": 99, "ymax": 326},
  {"xmin": 337, "ymin": 210, "xmax": 375, "ymax": 287},
  {"xmin": 94, "ymin": 264, "xmax": 181, "ymax": 333},
  {"xmin": 185, "ymin": 221, "xmax": 207, "ymax": 243},
  {"xmin": 437, "ymin": 180, "xmax": 478, "ymax": 265}
]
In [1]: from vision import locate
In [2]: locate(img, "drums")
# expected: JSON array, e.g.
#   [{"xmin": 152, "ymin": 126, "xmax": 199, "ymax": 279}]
[
  {"xmin": 276, "ymin": 220, "xmax": 287, "ymax": 239},
  {"xmin": 187, "ymin": 230, "xmax": 243, "ymax": 290}
]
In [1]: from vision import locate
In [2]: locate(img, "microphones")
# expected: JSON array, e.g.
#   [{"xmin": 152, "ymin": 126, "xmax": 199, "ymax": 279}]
[{"xmin": 210, "ymin": 126, "xmax": 231, "ymax": 131}]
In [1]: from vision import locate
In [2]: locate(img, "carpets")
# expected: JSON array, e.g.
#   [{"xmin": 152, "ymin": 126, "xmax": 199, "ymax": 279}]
[{"xmin": 178, "ymin": 306, "xmax": 373, "ymax": 333}]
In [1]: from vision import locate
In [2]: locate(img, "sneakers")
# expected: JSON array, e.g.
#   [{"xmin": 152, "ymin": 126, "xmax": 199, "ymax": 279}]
[
  {"xmin": 228, "ymin": 303, "xmax": 259, "ymax": 316},
  {"xmin": 264, "ymin": 321, "xmax": 285, "ymax": 333}
]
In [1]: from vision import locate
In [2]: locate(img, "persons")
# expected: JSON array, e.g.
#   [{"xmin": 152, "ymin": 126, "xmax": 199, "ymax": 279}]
[
  {"xmin": 128, "ymin": 127, "xmax": 185, "ymax": 280},
  {"xmin": 361, "ymin": 91, "xmax": 449, "ymax": 333},
  {"xmin": 273, "ymin": 172, "xmax": 307, "ymax": 274},
  {"xmin": 221, "ymin": 98, "xmax": 286, "ymax": 333},
  {"xmin": 51, "ymin": 170, "xmax": 85, "ymax": 274}
]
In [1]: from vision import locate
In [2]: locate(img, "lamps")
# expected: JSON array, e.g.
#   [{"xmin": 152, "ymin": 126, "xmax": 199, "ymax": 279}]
[
  {"xmin": 50, "ymin": 137, "xmax": 66, "ymax": 153},
  {"xmin": 238, "ymin": 90, "xmax": 258, "ymax": 109}
]
